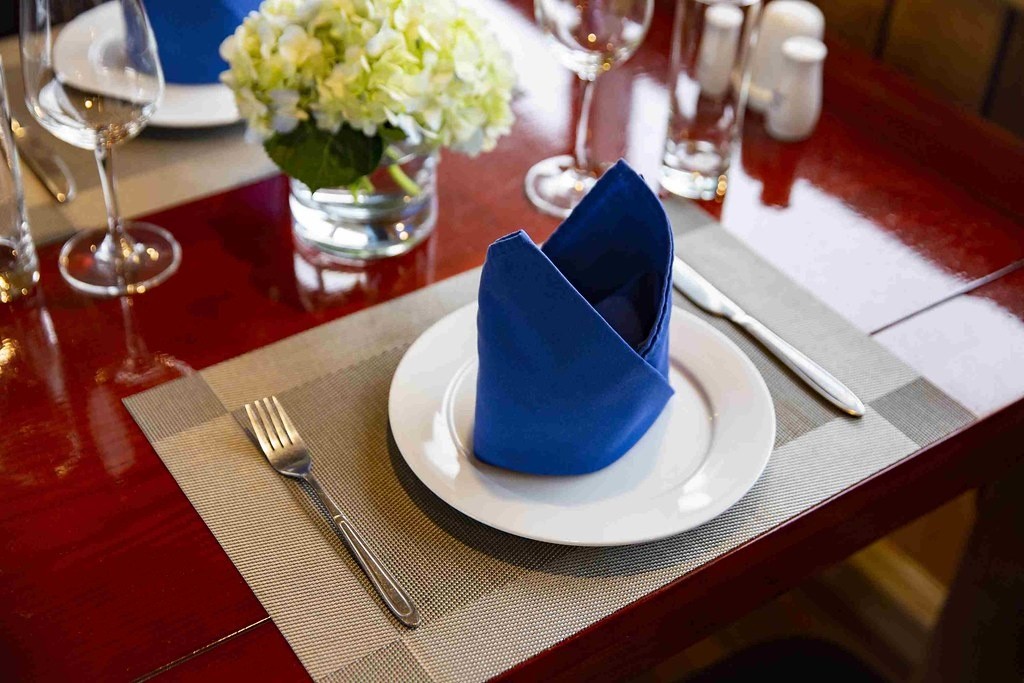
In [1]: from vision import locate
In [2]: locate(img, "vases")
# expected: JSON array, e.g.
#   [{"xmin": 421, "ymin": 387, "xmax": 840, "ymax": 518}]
[{"xmin": 286, "ymin": 133, "xmax": 441, "ymax": 260}]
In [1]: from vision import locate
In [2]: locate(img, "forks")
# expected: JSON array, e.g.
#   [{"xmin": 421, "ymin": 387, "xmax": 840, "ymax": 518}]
[{"xmin": 245, "ymin": 395, "xmax": 421, "ymax": 627}]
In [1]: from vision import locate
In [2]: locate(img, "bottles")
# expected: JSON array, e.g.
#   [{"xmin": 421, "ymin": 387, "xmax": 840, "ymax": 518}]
[
  {"xmin": 695, "ymin": 2, "xmax": 745, "ymax": 97},
  {"xmin": 766, "ymin": 36, "xmax": 828, "ymax": 142},
  {"xmin": 748, "ymin": 1, "xmax": 825, "ymax": 114}
]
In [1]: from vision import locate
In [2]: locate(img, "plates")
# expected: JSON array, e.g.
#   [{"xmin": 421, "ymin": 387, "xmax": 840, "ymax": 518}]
[
  {"xmin": 389, "ymin": 299, "xmax": 777, "ymax": 546},
  {"xmin": 53, "ymin": 1, "xmax": 241, "ymax": 127}
]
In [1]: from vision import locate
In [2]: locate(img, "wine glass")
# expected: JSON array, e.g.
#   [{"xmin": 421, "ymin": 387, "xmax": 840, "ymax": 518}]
[
  {"xmin": 18, "ymin": 1, "xmax": 181, "ymax": 295},
  {"xmin": 523, "ymin": 0, "xmax": 655, "ymax": 221}
]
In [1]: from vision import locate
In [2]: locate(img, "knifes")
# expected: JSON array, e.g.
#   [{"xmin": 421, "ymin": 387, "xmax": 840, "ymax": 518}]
[
  {"xmin": 671, "ymin": 255, "xmax": 865, "ymax": 418},
  {"xmin": 10, "ymin": 111, "xmax": 77, "ymax": 204}
]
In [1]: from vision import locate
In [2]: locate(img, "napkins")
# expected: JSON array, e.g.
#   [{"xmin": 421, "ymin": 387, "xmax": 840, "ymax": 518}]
[
  {"xmin": 120, "ymin": 0, "xmax": 262, "ymax": 84},
  {"xmin": 466, "ymin": 158, "xmax": 676, "ymax": 477}
]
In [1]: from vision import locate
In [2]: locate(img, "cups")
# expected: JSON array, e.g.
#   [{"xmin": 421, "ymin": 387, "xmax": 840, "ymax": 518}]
[
  {"xmin": 0, "ymin": 51, "xmax": 44, "ymax": 305},
  {"xmin": 656, "ymin": 0, "xmax": 764, "ymax": 200}
]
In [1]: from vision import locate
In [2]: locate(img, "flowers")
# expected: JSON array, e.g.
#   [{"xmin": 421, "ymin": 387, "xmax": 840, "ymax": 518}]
[{"xmin": 218, "ymin": 0, "xmax": 517, "ymax": 197}]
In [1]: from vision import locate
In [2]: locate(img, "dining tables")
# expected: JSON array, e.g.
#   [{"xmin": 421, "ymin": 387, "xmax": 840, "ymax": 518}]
[{"xmin": 0, "ymin": 0, "xmax": 1024, "ymax": 683}]
